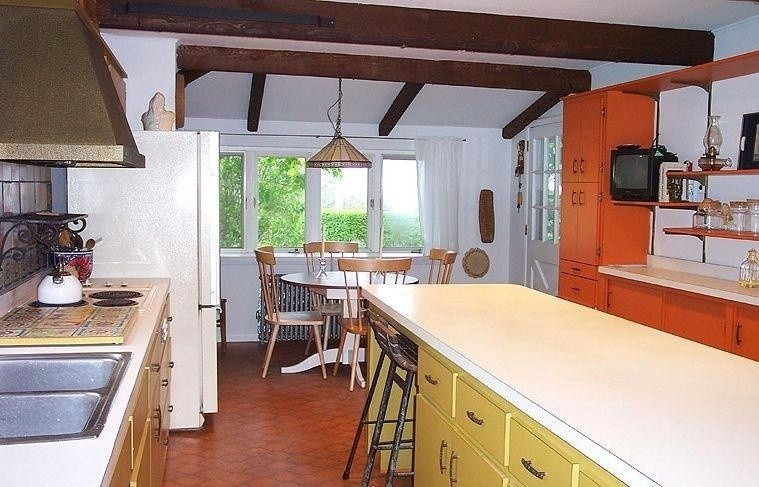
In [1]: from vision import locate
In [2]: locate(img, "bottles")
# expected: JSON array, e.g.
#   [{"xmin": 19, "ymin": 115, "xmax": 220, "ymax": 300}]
[
  {"xmin": 689, "ymin": 198, "xmax": 728, "ymax": 232},
  {"xmin": 727, "ymin": 200, "xmax": 746, "ymax": 234},
  {"xmin": 744, "ymin": 198, "xmax": 759, "ymax": 233},
  {"xmin": 737, "ymin": 249, "xmax": 759, "ymax": 288}
]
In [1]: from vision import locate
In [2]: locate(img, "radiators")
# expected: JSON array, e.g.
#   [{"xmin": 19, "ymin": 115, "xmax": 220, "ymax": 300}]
[{"xmin": 259, "ymin": 276, "xmax": 342, "ymax": 343}]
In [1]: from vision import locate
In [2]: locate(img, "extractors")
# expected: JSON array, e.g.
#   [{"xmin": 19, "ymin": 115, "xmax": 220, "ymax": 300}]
[{"xmin": 0, "ymin": 0, "xmax": 147, "ymax": 170}]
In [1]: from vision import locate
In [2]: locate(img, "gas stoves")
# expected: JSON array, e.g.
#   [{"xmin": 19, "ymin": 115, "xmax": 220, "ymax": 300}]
[{"xmin": 26, "ymin": 288, "xmax": 159, "ymax": 316}]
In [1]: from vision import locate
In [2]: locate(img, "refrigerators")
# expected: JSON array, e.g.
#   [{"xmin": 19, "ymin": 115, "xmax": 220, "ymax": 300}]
[{"xmin": 65, "ymin": 129, "xmax": 223, "ymax": 431}]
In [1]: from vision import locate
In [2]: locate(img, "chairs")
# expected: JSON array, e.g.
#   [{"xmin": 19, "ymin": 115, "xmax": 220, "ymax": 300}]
[
  {"xmin": 254, "ymin": 245, "xmax": 328, "ymax": 380},
  {"xmin": 302, "ymin": 240, "xmax": 360, "ymax": 357},
  {"xmin": 342, "ymin": 315, "xmax": 417, "ymax": 487},
  {"xmin": 426, "ymin": 247, "xmax": 457, "ymax": 285},
  {"xmin": 333, "ymin": 258, "xmax": 412, "ymax": 391}
]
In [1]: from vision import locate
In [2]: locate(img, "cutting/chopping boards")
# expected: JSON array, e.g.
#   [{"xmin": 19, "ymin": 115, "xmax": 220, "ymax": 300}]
[{"xmin": 0, "ymin": 307, "xmax": 141, "ymax": 346}]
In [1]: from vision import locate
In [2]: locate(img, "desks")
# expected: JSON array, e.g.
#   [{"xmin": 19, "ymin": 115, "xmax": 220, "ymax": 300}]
[{"xmin": 281, "ymin": 270, "xmax": 418, "ymax": 385}]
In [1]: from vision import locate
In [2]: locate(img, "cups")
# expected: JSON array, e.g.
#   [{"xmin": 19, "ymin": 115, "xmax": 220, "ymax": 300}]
[{"xmin": 667, "ymin": 171, "xmax": 684, "ymax": 204}]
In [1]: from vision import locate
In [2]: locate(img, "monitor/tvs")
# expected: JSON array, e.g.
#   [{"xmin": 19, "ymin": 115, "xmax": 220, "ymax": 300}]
[{"xmin": 610, "ymin": 149, "xmax": 678, "ymax": 201}]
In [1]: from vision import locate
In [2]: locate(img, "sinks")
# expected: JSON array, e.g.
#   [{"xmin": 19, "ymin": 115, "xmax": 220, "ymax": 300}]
[
  {"xmin": 0, "ymin": 392, "xmax": 115, "ymax": 445},
  {"xmin": 0, "ymin": 351, "xmax": 132, "ymax": 394}
]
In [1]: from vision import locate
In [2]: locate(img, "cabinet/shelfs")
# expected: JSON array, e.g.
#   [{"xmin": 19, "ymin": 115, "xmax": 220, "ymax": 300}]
[
  {"xmin": 603, "ymin": 275, "xmax": 663, "ymax": 331},
  {"xmin": 505, "ymin": 419, "xmax": 628, "ymax": 487},
  {"xmin": 559, "ymin": 183, "xmax": 651, "ymax": 265},
  {"xmin": 558, "ymin": 88, "xmax": 656, "ymax": 182},
  {"xmin": 663, "ymin": 286, "xmax": 758, "ymax": 362},
  {"xmin": 557, "ymin": 258, "xmax": 601, "ymax": 309},
  {"xmin": 613, "ymin": 166, "xmax": 759, "ymax": 243},
  {"xmin": 130, "ymin": 338, "xmax": 159, "ymax": 486},
  {"xmin": 157, "ymin": 294, "xmax": 174, "ymax": 487},
  {"xmin": 410, "ymin": 347, "xmax": 508, "ymax": 486},
  {"xmin": 104, "ymin": 422, "xmax": 134, "ymax": 487}
]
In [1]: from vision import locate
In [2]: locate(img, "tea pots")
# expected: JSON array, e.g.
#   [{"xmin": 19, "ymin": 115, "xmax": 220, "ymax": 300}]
[{"xmin": 33, "ymin": 255, "xmax": 84, "ymax": 306}]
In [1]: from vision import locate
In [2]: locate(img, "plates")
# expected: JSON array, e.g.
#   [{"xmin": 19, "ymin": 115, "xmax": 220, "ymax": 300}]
[
  {"xmin": 461, "ymin": 247, "xmax": 491, "ymax": 280},
  {"xmin": 615, "ymin": 143, "xmax": 641, "ymax": 151}
]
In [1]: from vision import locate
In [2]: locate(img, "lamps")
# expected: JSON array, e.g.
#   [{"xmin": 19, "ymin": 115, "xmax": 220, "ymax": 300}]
[{"xmin": 306, "ymin": 76, "xmax": 371, "ymax": 169}]
